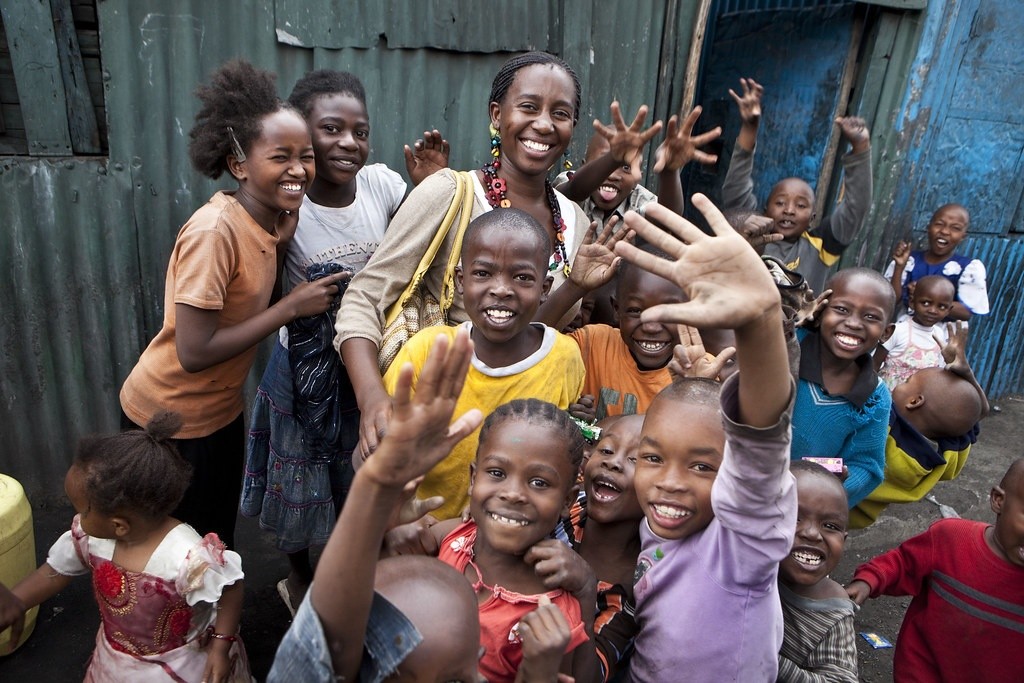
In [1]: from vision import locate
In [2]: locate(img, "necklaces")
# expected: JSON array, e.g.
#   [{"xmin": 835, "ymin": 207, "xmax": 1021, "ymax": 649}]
[{"xmin": 482, "ymin": 162, "xmax": 572, "ymax": 277}]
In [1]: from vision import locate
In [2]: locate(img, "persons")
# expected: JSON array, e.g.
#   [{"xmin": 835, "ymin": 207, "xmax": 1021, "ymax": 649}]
[
  {"xmin": 0, "ymin": 411, "xmax": 250, "ymax": 683},
  {"xmin": 118, "ymin": 61, "xmax": 351, "ymax": 550},
  {"xmin": 268, "ymin": 50, "xmax": 989, "ymax": 683},
  {"xmin": 237, "ymin": 70, "xmax": 450, "ymax": 620},
  {"xmin": 842, "ymin": 458, "xmax": 1024, "ymax": 682}
]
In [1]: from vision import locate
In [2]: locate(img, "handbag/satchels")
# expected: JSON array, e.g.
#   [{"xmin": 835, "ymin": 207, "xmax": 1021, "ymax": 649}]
[{"xmin": 378, "ymin": 168, "xmax": 473, "ymax": 374}]
